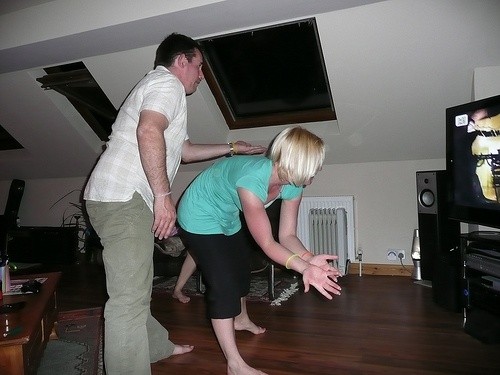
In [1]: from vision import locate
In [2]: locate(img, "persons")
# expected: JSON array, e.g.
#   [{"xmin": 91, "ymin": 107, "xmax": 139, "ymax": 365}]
[
  {"xmin": 172, "ymin": 254, "xmax": 197, "ymax": 303},
  {"xmin": 85, "ymin": 31, "xmax": 266, "ymax": 375},
  {"xmin": 178, "ymin": 125, "xmax": 342, "ymax": 375}
]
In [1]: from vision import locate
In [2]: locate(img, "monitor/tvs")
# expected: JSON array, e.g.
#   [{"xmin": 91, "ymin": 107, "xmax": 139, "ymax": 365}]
[{"xmin": 446, "ymin": 95, "xmax": 500, "ymax": 244}]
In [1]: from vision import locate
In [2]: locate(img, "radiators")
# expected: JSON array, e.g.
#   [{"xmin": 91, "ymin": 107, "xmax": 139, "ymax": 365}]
[
  {"xmin": 297, "ymin": 196, "xmax": 355, "ymax": 261},
  {"xmin": 308, "ymin": 208, "xmax": 348, "ymax": 276}
]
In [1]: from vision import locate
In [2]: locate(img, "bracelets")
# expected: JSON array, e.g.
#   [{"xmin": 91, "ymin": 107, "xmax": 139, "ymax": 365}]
[
  {"xmin": 152, "ymin": 191, "xmax": 173, "ymax": 197},
  {"xmin": 228, "ymin": 142, "xmax": 235, "ymax": 158},
  {"xmin": 285, "ymin": 253, "xmax": 299, "ymax": 269}
]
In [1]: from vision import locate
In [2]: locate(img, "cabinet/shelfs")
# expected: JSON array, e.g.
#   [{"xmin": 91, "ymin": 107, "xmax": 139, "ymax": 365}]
[{"xmin": 457, "ymin": 231, "xmax": 500, "ymax": 330}]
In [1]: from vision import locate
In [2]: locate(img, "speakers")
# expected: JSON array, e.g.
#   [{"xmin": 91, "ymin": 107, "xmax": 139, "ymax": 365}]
[{"xmin": 415, "ymin": 170, "xmax": 461, "ymax": 282}]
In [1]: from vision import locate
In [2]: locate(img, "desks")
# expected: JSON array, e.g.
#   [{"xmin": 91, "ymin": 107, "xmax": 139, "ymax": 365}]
[{"xmin": 0, "ymin": 272, "xmax": 62, "ymax": 375}]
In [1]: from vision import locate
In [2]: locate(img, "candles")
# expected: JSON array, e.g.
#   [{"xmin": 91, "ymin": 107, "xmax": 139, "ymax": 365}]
[
  {"xmin": 2, "ymin": 266, "xmax": 6, "ymax": 293},
  {"xmin": 6, "ymin": 270, "xmax": 10, "ymax": 292}
]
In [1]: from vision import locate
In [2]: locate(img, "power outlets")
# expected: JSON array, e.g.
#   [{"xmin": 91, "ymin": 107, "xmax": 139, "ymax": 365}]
[{"xmin": 387, "ymin": 249, "xmax": 405, "ymax": 260}]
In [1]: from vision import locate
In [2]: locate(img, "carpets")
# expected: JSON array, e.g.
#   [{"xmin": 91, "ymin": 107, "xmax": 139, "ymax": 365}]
[
  {"xmin": 36, "ymin": 306, "xmax": 104, "ymax": 375},
  {"xmin": 153, "ymin": 269, "xmax": 304, "ymax": 311}
]
